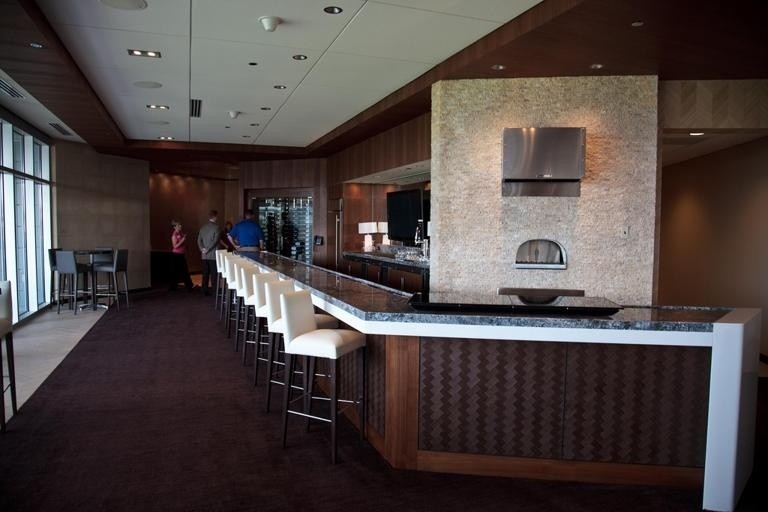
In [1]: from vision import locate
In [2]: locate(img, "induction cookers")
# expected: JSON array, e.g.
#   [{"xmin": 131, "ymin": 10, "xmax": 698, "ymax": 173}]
[{"xmin": 406, "ymin": 290, "xmax": 624, "ymax": 317}]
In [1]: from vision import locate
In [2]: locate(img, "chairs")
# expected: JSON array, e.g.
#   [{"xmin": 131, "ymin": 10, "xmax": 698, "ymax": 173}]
[
  {"xmin": 45, "ymin": 236, "xmax": 133, "ymax": 315},
  {"xmin": 209, "ymin": 247, "xmax": 373, "ymax": 467},
  {"xmin": 1, "ymin": 281, "xmax": 23, "ymax": 433}
]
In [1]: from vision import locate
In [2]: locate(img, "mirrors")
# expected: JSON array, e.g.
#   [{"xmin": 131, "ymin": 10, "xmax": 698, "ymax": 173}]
[{"xmin": 371, "ymin": 171, "xmax": 431, "ymax": 249}]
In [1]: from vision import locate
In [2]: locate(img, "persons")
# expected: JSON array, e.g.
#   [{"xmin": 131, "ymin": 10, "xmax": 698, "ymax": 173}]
[
  {"xmin": 171, "ymin": 218, "xmax": 199, "ymax": 290},
  {"xmin": 266, "ymin": 215, "xmax": 278, "ymax": 253},
  {"xmin": 281, "ymin": 211, "xmax": 299, "ymax": 258},
  {"xmin": 197, "ymin": 209, "xmax": 222, "ymax": 292},
  {"xmin": 219, "ymin": 222, "xmax": 233, "ymax": 249},
  {"xmin": 227, "ymin": 209, "xmax": 264, "ymax": 252}
]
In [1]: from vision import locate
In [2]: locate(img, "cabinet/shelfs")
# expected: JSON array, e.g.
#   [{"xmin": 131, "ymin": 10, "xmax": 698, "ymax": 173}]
[{"xmin": 342, "ymin": 253, "xmax": 429, "ymax": 296}]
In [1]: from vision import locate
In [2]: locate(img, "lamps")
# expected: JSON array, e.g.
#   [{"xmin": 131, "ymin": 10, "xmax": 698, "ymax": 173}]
[
  {"xmin": 376, "ymin": 221, "xmax": 391, "ymax": 245},
  {"xmin": 356, "ymin": 219, "xmax": 378, "ymax": 254}
]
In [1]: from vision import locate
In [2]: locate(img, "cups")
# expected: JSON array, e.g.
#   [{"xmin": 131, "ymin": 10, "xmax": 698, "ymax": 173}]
[{"xmin": 392, "ymin": 248, "xmax": 418, "ymax": 261}]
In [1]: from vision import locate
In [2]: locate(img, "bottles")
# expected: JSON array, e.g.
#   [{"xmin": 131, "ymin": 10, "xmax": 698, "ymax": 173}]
[{"xmin": 287, "ymin": 241, "xmax": 305, "ymax": 260}]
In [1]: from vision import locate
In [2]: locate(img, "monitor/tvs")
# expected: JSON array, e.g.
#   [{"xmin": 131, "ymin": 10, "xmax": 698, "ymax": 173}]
[{"xmin": 385, "ymin": 188, "xmax": 425, "ymax": 244}]
